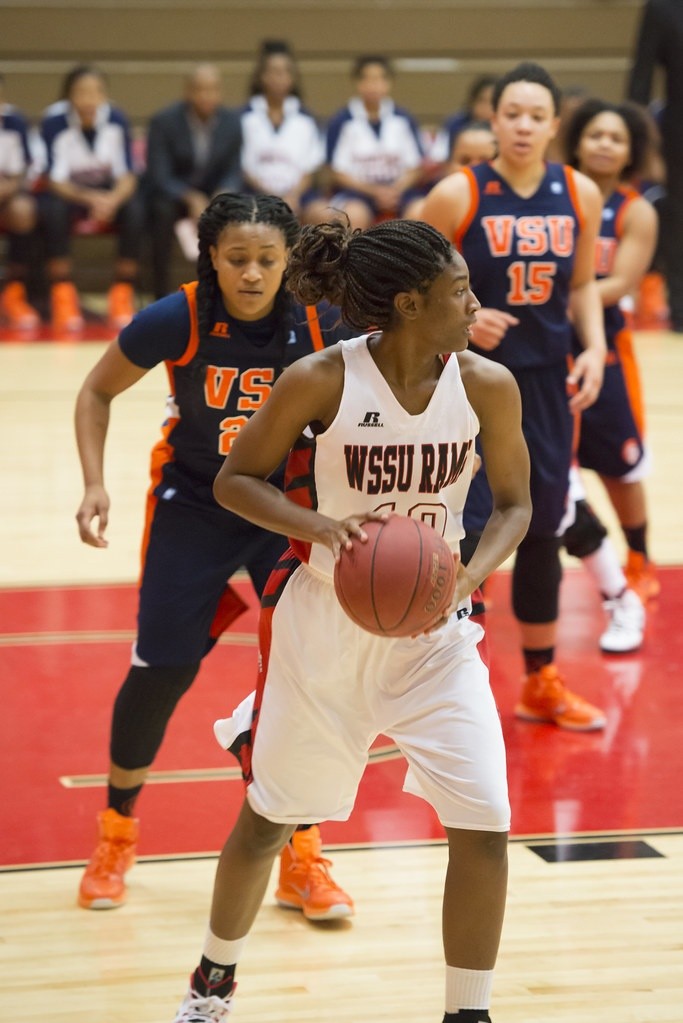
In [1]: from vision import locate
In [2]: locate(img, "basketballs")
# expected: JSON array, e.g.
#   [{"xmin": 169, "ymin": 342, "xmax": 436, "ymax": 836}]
[{"xmin": 333, "ymin": 515, "xmax": 458, "ymax": 639}]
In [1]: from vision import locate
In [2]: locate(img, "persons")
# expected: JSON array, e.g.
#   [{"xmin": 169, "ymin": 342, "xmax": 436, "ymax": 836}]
[
  {"xmin": 2, "ymin": 43, "xmax": 683, "ymax": 732},
  {"xmin": 73, "ymin": 193, "xmax": 354, "ymax": 924},
  {"xmin": 169, "ymin": 221, "xmax": 532, "ymax": 1023}
]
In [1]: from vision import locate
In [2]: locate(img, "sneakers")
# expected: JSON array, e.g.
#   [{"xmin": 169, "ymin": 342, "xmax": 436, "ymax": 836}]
[
  {"xmin": 50, "ymin": 282, "xmax": 84, "ymax": 331},
  {"xmin": 513, "ymin": 664, "xmax": 606, "ymax": 730},
  {"xmin": 2, "ymin": 282, "xmax": 40, "ymax": 330},
  {"xmin": 598, "ymin": 587, "xmax": 646, "ymax": 651},
  {"xmin": 625, "ymin": 551, "xmax": 659, "ymax": 598},
  {"xmin": 173, "ymin": 967, "xmax": 238, "ymax": 1023},
  {"xmin": 442, "ymin": 1008, "xmax": 492, "ymax": 1023},
  {"xmin": 275, "ymin": 826, "xmax": 354, "ymax": 920},
  {"xmin": 107, "ymin": 282, "xmax": 134, "ymax": 329},
  {"xmin": 77, "ymin": 809, "xmax": 140, "ymax": 909}
]
